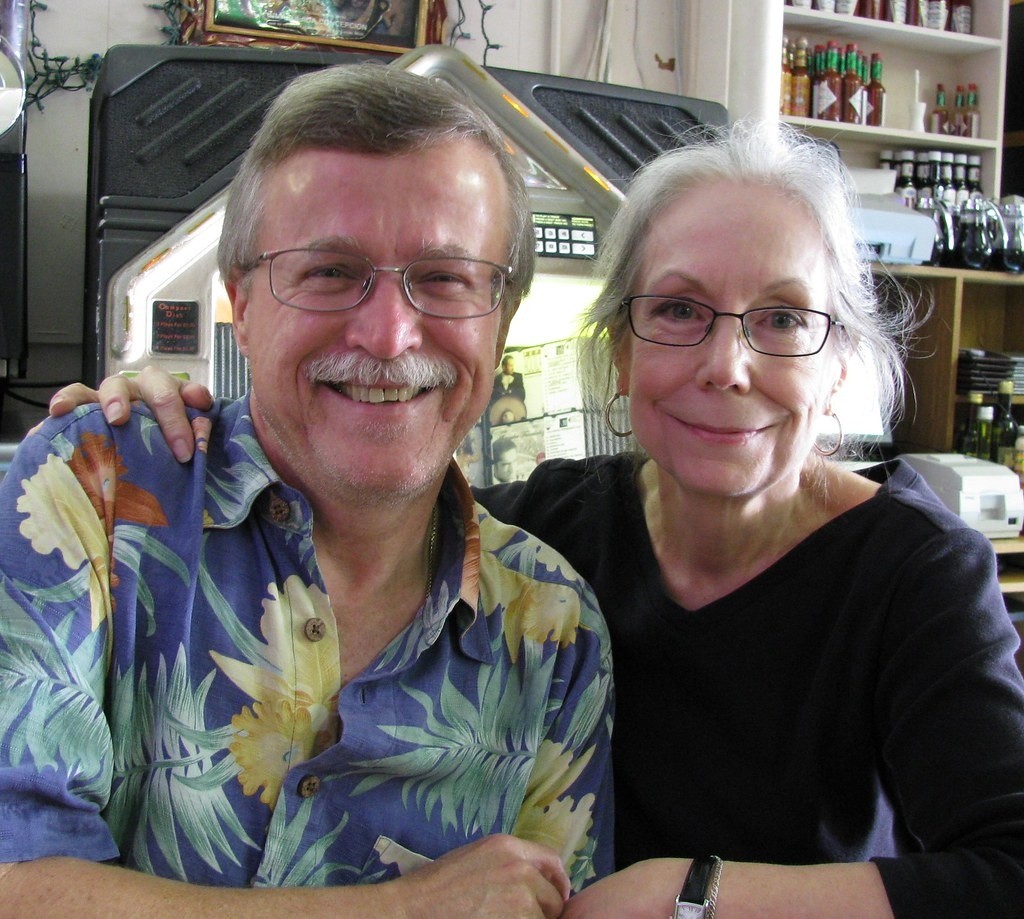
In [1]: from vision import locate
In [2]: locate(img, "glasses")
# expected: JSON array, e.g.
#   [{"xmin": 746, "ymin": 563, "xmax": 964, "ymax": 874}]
[
  {"xmin": 617, "ymin": 295, "xmax": 845, "ymax": 357},
  {"xmin": 239, "ymin": 248, "xmax": 512, "ymax": 319}
]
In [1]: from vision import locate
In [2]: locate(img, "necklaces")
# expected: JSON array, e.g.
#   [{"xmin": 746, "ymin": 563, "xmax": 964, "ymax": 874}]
[{"xmin": 425, "ymin": 507, "xmax": 436, "ymax": 602}]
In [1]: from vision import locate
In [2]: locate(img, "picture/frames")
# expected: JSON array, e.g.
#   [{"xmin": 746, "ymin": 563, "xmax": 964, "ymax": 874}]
[{"xmin": 180, "ymin": 0, "xmax": 448, "ymax": 58}]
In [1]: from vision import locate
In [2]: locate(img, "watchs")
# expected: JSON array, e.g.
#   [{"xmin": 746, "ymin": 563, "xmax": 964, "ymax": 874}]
[{"xmin": 669, "ymin": 855, "xmax": 723, "ymax": 919}]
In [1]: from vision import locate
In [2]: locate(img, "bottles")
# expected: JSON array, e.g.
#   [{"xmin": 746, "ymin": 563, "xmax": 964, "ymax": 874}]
[
  {"xmin": 779, "ymin": 36, "xmax": 887, "ymax": 126},
  {"xmin": 958, "ymin": 391, "xmax": 983, "ymax": 460},
  {"xmin": 928, "ymin": 84, "xmax": 949, "ymax": 134},
  {"xmin": 990, "ymin": 380, "xmax": 1018, "ymax": 471},
  {"xmin": 878, "ymin": 149, "xmax": 984, "ymax": 213},
  {"xmin": 976, "ymin": 405, "xmax": 993, "ymax": 462},
  {"xmin": 786, "ymin": 0, "xmax": 972, "ymax": 36},
  {"xmin": 961, "ymin": 83, "xmax": 980, "ymax": 138},
  {"xmin": 1013, "ymin": 425, "xmax": 1024, "ymax": 493},
  {"xmin": 949, "ymin": 84, "xmax": 965, "ymax": 135}
]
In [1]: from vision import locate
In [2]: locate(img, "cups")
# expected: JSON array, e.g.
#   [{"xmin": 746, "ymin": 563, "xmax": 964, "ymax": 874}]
[{"xmin": 909, "ymin": 102, "xmax": 927, "ymax": 133}]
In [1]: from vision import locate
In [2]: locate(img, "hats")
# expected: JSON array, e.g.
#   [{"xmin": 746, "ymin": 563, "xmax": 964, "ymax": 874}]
[{"xmin": 490, "ymin": 395, "xmax": 527, "ymax": 426}]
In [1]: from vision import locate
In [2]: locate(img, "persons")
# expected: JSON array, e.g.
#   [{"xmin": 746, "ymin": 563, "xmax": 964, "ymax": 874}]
[
  {"xmin": 0, "ymin": 61, "xmax": 615, "ymax": 919},
  {"xmin": 492, "ymin": 437, "xmax": 530, "ymax": 484},
  {"xmin": 49, "ymin": 118, "xmax": 1024, "ymax": 919},
  {"xmin": 491, "ymin": 356, "xmax": 526, "ymax": 403}
]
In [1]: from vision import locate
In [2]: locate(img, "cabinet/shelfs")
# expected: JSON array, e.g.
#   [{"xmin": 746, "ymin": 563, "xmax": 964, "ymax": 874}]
[
  {"xmin": 870, "ymin": 262, "xmax": 1024, "ymax": 453},
  {"xmin": 679, "ymin": 0, "xmax": 1010, "ymax": 205},
  {"xmin": 991, "ymin": 534, "xmax": 1024, "ymax": 623}
]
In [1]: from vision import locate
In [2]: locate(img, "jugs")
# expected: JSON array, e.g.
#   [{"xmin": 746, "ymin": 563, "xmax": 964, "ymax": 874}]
[{"xmin": 914, "ymin": 196, "xmax": 1024, "ymax": 275}]
